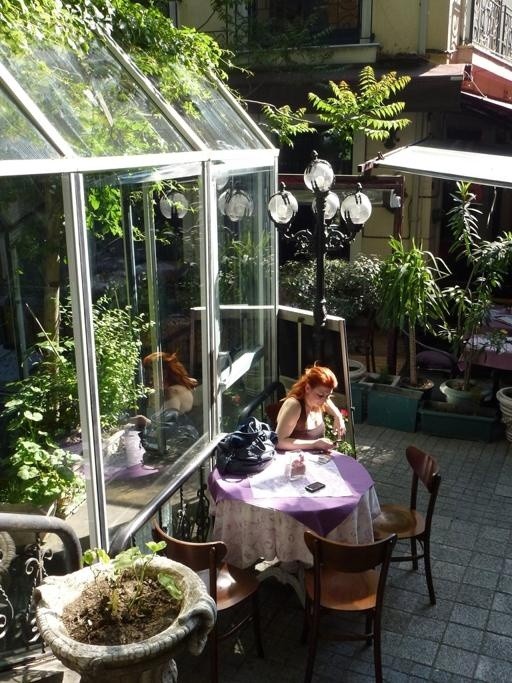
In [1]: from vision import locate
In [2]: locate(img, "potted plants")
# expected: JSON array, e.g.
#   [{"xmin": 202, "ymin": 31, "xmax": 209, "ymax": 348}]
[{"xmin": 32, "ymin": 539, "xmax": 218, "ymax": 683}]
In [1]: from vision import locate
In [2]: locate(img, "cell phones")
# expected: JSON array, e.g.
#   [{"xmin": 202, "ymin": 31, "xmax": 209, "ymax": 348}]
[
  {"xmin": 305, "ymin": 481, "xmax": 325, "ymax": 492},
  {"xmin": 333, "ymin": 439, "xmax": 343, "ymax": 445}
]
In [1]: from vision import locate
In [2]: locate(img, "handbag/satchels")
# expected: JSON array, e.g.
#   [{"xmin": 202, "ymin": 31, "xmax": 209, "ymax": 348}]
[{"xmin": 216, "ymin": 417, "xmax": 278, "ymax": 476}]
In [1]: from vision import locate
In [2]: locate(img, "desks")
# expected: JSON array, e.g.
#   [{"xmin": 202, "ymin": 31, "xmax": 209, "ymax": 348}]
[{"xmin": 208, "ymin": 448, "xmax": 381, "ymax": 616}]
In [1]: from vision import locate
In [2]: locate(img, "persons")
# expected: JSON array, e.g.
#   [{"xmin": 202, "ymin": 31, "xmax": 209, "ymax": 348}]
[
  {"xmin": 276, "ymin": 358, "xmax": 347, "ymax": 457},
  {"xmin": 126, "ymin": 351, "xmax": 199, "ymax": 430}
]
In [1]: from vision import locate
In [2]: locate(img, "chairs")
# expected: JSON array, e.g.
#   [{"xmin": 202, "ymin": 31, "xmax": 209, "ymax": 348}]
[
  {"xmin": 360, "ymin": 445, "xmax": 441, "ymax": 614},
  {"xmin": 266, "ymin": 401, "xmax": 294, "ymax": 438},
  {"xmin": 301, "ymin": 531, "xmax": 397, "ymax": 683},
  {"xmin": 154, "ymin": 520, "xmax": 264, "ymax": 683}
]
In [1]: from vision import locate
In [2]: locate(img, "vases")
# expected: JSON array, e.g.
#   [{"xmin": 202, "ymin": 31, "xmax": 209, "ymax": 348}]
[
  {"xmin": 418, "ymin": 401, "xmax": 500, "ymax": 444},
  {"xmin": 496, "ymin": 387, "xmax": 512, "ymax": 443},
  {"xmin": 351, "ymin": 382, "xmax": 366, "ymax": 425},
  {"xmin": 369, "ymin": 384, "xmax": 423, "ymax": 433}
]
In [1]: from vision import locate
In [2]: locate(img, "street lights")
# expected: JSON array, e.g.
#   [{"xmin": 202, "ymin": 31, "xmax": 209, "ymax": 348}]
[
  {"xmin": 158, "ymin": 161, "xmax": 256, "ymax": 397},
  {"xmin": 269, "ymin": 144, "xmax": 374, "ymax": 363}
]
